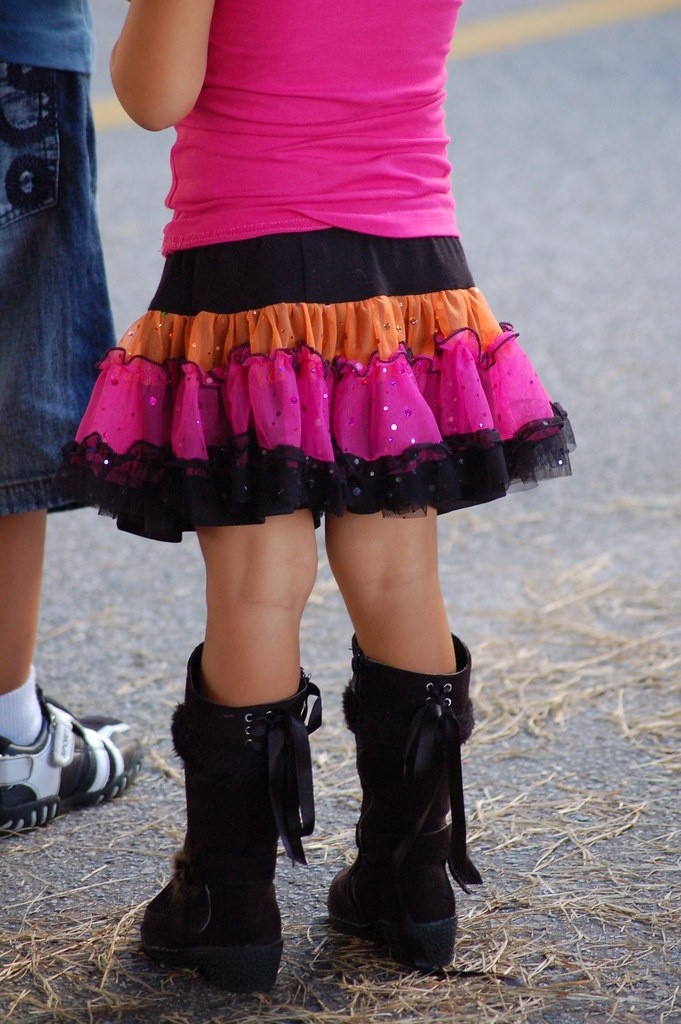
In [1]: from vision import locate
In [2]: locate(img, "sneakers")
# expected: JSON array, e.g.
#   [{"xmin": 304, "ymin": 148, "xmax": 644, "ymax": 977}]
[{"xmin": 0, "ymin": 682, "xmax": 143, "ymax": 836}]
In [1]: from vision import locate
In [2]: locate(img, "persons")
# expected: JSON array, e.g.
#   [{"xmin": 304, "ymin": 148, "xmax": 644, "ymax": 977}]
[
  {"xmin": 49, "ymin": 0, "xmax": 580, "ymax": 995},
  {"xmin": 1, "ymin": 1, "xmax": 143, "ymax": 832}
]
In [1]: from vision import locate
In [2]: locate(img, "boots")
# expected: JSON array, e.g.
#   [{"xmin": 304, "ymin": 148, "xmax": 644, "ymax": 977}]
[
  {"xmin": 327, "ymin": 634, "xmax": 483, "ymax": 966},
  {"xmin": 139, "ymin": 642, "xmax": 322, "ymax": 992}
]
